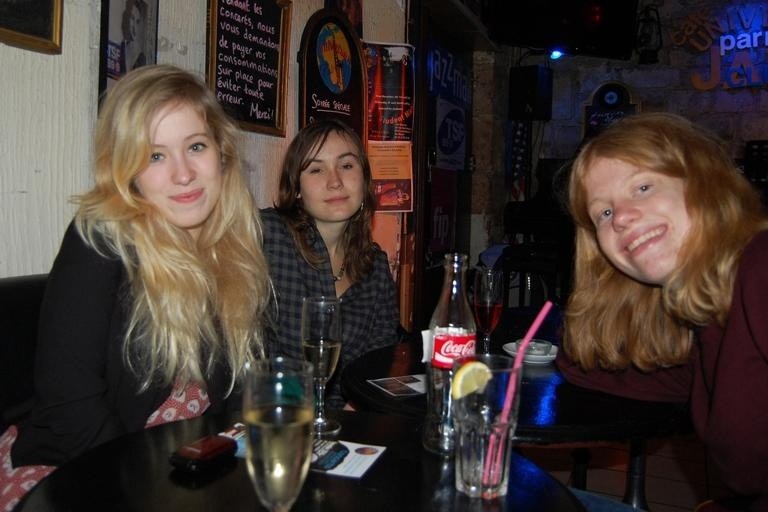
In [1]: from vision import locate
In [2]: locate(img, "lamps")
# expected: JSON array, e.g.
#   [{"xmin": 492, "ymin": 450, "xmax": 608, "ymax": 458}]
[{"xmin": 514, "ymin": 47, "xmax": 566, "ymax": 67}]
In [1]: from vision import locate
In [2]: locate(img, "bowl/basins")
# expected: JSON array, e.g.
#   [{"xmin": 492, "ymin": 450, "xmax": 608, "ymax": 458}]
[{"xmin": 516, "ymin": 336, "xmax": 553, "ymax": 355}]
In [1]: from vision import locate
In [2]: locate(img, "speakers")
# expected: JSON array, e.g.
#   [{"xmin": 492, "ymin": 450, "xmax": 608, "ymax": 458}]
[{"xmin": 508, "ymin": 64, "xmax": 553, "ymax": 121}]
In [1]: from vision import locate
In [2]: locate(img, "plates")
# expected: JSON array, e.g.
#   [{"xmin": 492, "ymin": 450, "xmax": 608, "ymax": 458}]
[{"xmin": 501, "ymin": 341, "xmax": 559, "ymax": 364}]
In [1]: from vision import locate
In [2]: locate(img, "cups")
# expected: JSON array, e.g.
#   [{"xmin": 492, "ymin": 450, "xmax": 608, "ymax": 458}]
[{"xmin": 448, "ymin": 350, "xmax": 522, "ymax": 501}]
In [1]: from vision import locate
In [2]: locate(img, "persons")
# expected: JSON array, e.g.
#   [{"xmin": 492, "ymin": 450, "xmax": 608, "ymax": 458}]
[
  {"xmin": 119, "ymin": 0, "xmax": 148, "ymax": 75},
  {"xmin": 533, "ymin": 107, "xmax": 766, "ymax": 511},
  {"xmin": 0, "ymin": 63, "xmax": 284, "ymax": 512},
  {"xmin": 248, "ymin": 116, "xmax": 417, "ymax": 414}
]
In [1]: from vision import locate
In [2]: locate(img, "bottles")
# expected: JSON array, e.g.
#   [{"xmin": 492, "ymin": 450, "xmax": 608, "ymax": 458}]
[{"xmin": 419, "ymin": 250, "xmax": 481, "ymax": 458}]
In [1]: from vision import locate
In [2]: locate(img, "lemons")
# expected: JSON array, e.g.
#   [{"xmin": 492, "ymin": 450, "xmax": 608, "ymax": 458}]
[{"xmin": 452, "ymin": 361, "xmax": 493, "ymax": 400}]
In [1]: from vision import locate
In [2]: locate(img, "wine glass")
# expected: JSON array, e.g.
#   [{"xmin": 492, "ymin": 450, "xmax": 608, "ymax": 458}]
[
  {"xmin": 239, "ymin": 355, "xmax": 315, "ymax": 510},
  {"xmin": 472, "ymin": 269, "xmax": 505, "ymax": 353},
  {"xmin": 300, "ymin": 292, "xmax": 346, "ymax": 438}
]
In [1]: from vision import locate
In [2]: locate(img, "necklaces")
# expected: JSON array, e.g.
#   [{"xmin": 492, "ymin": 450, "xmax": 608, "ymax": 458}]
[{"xmin": 331, "ymin": 252, "xmax": 349, "ymax": 287}]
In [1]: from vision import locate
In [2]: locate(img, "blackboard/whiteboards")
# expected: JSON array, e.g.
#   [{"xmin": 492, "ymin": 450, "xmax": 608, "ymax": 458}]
[
  {"xmin": 0, "ymin": 0, "xmax": 63, "ymax": 56},
  {"xmin": 205, "ymin": 0, "xmax": 293, "ymax": 138},
  {"xmin": 578, "ymin": 79, "xmax": 642, "ymax": 146}
]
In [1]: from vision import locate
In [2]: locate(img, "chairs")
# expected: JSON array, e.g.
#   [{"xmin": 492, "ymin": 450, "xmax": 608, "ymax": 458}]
[{"xmin": 502, "ymin": 200, "xmax": 533, "ymax": 311}]
